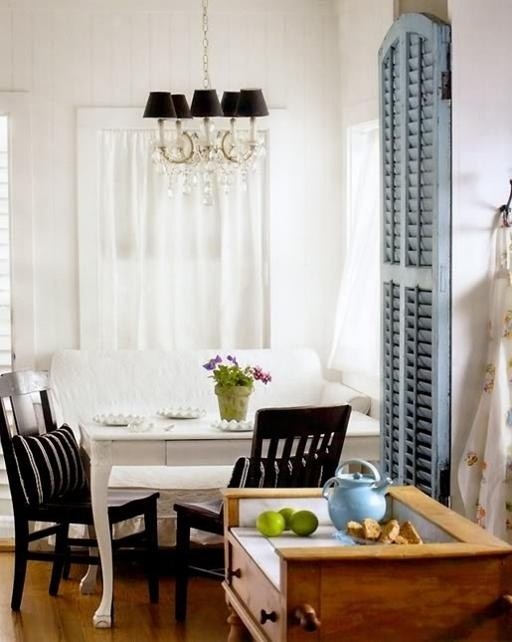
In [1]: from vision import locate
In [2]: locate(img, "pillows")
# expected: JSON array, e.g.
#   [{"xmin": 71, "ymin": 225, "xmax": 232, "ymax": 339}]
[
  {"xmin": 221, "ymin": 446, "xmax": 335, "ymax": 517},
  {"xmin": 12, "ymin": 423, "xmax": 91, "ymax": 506}
]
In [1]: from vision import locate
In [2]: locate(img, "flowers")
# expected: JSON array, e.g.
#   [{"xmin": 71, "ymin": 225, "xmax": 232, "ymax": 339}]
[{"xmin": 202, "ymin": 354, "xmax": 273, "ymax": 388}]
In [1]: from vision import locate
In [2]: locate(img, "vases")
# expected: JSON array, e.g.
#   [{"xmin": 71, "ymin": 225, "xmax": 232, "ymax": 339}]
[{"xmin": 215, "ymin": 385, "xmax": 253, "ymax": 423}]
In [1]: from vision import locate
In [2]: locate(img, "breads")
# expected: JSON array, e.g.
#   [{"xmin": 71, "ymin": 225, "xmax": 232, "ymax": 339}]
[{"xmin": 347, "ymin": 518, "xmax": 423, "ymax": 545}]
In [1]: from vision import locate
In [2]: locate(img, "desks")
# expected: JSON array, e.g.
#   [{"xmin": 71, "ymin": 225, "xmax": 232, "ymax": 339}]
[
  {"xmin": 77, "ymin": 419, "xmax": 380, "ymax": 627},
  {"xmin": 224, "ymin": 483, "xmax": 512, "ymax": 642}
]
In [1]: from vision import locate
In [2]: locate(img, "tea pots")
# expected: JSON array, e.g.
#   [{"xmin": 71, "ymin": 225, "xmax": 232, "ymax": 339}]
[{"xmin": 322, "ymin": 458, "xmax": 393, "ymax": 532}]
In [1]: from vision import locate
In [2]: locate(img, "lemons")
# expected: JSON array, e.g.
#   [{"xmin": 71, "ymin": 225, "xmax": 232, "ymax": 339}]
[
  {"xmin": 256, "ymin": 510, "xmax": 285, "ymax": 537},
  {"xmin": 278, "ymin": 507, "xmax": 294, "ymax": 530},
  {"xmin": 288, "ymin": 511, "xmax": 319, "ymax": 536}
]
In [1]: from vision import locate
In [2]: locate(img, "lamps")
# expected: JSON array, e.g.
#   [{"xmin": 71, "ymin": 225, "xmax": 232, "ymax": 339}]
[{"xmin": 141, "ymin": 1, "xmax": 272, "ymax": 215}]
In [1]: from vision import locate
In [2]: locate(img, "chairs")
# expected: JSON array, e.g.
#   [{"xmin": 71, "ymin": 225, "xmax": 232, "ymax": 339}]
[
  {"xmin": 172, "ymin": 406, "xmax": 352, "ymax": 622},
  {"xmin": 1, "ymin": 369, "xmax": 161, "ymax": 612}
]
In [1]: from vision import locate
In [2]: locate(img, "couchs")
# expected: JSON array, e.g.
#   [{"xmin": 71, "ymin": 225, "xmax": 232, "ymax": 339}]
[{"xmin": 47, "ymin": 345, "xmax": 373, "ymax": 520}]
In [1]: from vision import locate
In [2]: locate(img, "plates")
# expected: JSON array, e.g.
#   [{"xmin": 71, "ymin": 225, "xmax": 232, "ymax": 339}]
[
  {"xmin": 92, "ymin": 413, "xmax": 147, "ymax": 426},
  {"xmin": 156, "ymin": 406, "xmax": 207, "ymax": 419},
  {"xmin": 209, "ymin": 419, "xmax": 256, "ymax": 433}
]
